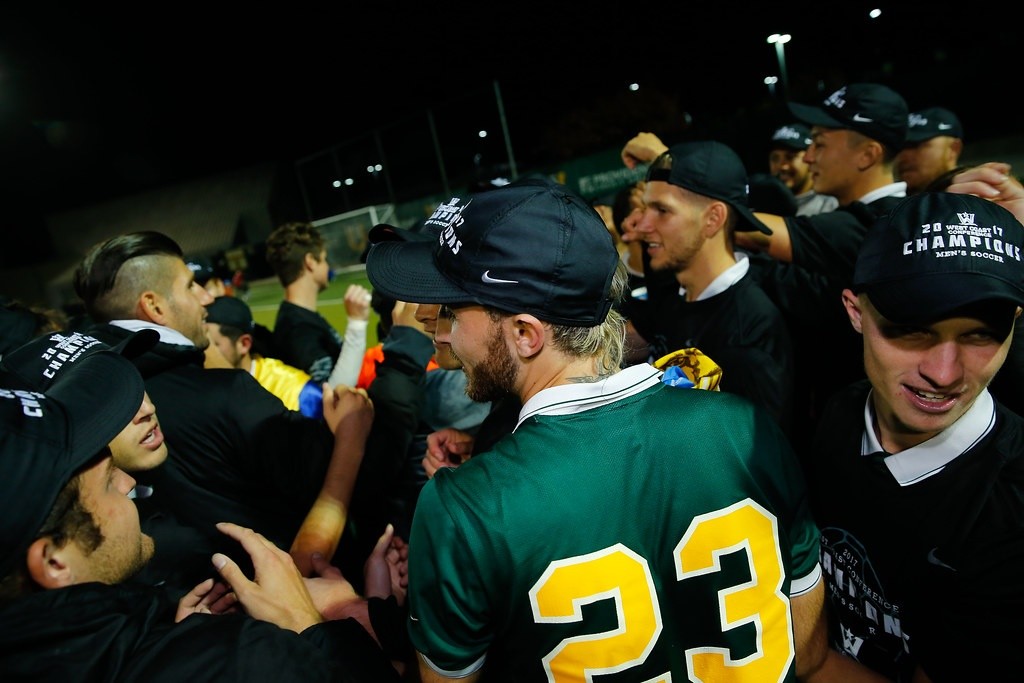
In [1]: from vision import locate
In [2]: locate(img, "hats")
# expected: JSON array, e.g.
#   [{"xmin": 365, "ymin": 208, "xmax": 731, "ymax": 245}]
[
  {"xmin": 852, "ymin": 192, "xmax": 1024, "ymax": 333},
  {"xmin": 0, "ymin": 350, "xmax": 146, "ymax": 555},
  {"xmin": 766, "ymin": 124, "xmax": 814, "ymax": 150},
  {"xmin": 786, "ymin": 80, "xmax": 911, "ymax": 151},
  {"xmin": 366, "ymin": 179, "xmax": 622, "ymax": 329},
  {"xmin": 0, "ymin": 329, "xmax": 160, "ymax": 395},
  {"xmin": 646, "ymin": 140, "xmax": 774, "ymax": 235},
  {"xmin": 902, "ymin": 105, "xmax": 962, "ymax": 148},
  {"xmin": 182, "ymin": 257, "xmax": 236, "ymax": 281},
  {"xmin": 368, "ymin": 190, "xmax": 483, "ymax": 247}
]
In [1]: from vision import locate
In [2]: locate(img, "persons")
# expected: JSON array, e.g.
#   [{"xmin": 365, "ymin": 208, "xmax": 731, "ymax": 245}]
[
  {"xmin": 1, "ymin": 350, "xmax": 404, "ymax": 682},
  {"xmin": 365, "ymin": 183, "xmax": 828, "ymax": 683},
  {"xmin": 787, "ymin": 189, "xmax": 1023, "ymax": 683},
  {"xmin": 0, "ymin": 81, "xmax": 1024, "ymax": 683}
]
